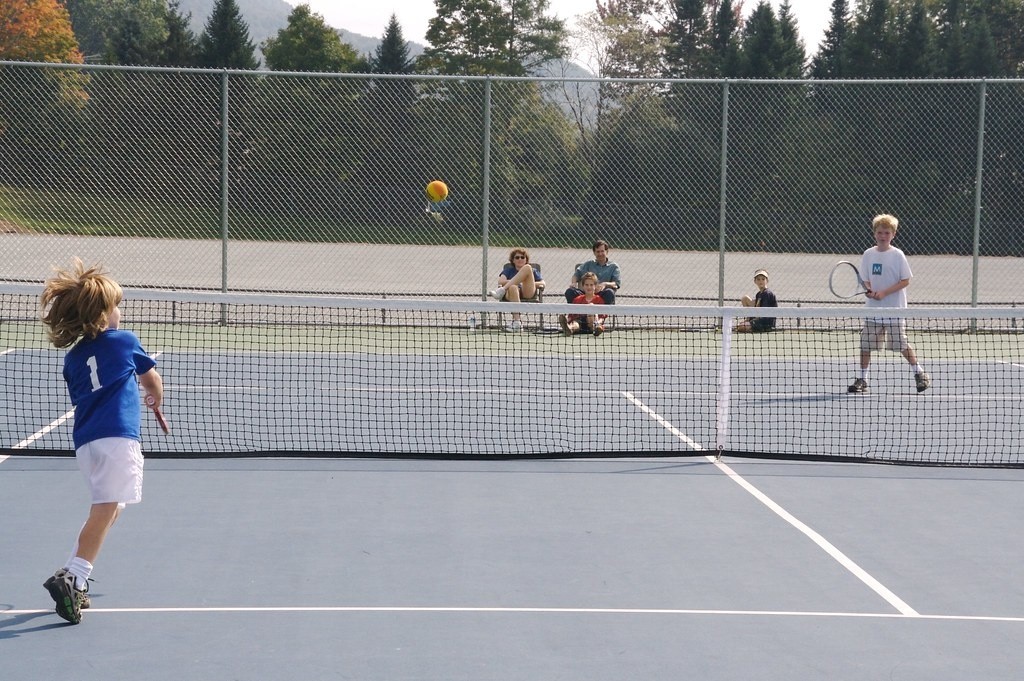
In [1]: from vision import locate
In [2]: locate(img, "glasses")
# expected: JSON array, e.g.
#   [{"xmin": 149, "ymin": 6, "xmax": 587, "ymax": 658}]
[{"xmin": 514, "ymin": 256, "xmax": 525, "ymax": 259}]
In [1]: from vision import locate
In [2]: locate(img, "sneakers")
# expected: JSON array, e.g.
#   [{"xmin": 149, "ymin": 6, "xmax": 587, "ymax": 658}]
[
  {"xmin": 593, "ymin": 326, "xmax": 604, "ymax": 336},
  {"xmin": 558, "ymin": 313, "xmax": 573, "ymax": 336},
  {"xmin": 847, "ymin": 378, "xmax": 868, "ymax": 392},
  {"xmin": 914, "ymin": 372, "xmax": 930, "ymax": 392},
  {"xmin": 504, "ymin": 321, "xmax": 524, "ymax": 332},
  {"xmin": 489, "ymin": 286, "xmax": 506, "ymax": 301},
  {"xmin": 42, "ymin": 568, "xmax": 90, "ymax": 624}
]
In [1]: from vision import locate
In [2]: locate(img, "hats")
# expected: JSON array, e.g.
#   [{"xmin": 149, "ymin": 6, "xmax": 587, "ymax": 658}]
[{"xmin": 754, "ymin": 270, "xmax": 768, "ymax": 278}]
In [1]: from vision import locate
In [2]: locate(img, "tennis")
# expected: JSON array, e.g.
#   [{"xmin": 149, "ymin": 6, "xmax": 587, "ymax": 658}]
[{"xmin": 425, "ymin": 180, "xmax": 449, "ymax": 202}]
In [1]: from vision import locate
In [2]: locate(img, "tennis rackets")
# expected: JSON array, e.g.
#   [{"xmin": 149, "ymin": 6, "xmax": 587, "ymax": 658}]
[
  {"xmin": 828, "ymin": 261, "xmax": 872, "ymax": 299},
  {"xmin": 146, "ymin": 394, "xmax": 169, "ymax": 435}
]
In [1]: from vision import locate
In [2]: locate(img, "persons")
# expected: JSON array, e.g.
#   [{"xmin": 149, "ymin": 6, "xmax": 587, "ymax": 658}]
[
  {"xmin": 736, "ymin": 268, "xmax": 778, "ymax": 333},
  {"xmin": 34, "ymin": 256, "xmax": 163, "ymax": 624},
  {"xmin": 558, "ymin": 271, "xmax": 608, "ymax": 336},
  {"xmin": 847, "ymin": 213, "xmax": 930, "ymax": 394},
  {"xmin": 564, "ymin": 240, "xmax": 622, "ymax": 306},
  {"xmin": 489, "ymin": 248, "xmax": 546, "ymax": 332}
]
